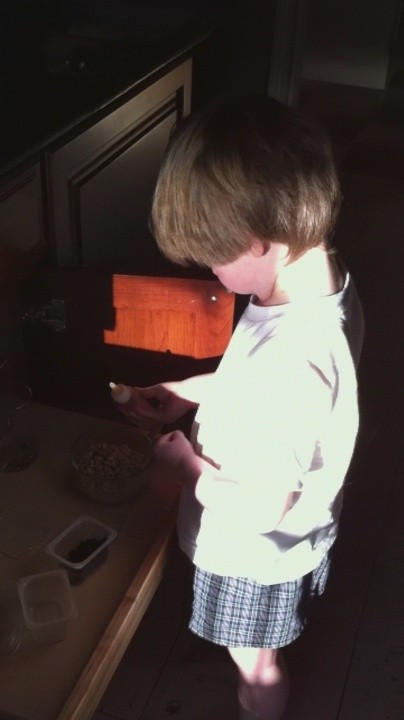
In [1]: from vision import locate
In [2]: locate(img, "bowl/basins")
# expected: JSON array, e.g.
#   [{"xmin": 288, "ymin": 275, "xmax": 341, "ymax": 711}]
[
  {"xmin": 15, "ymin": 568, "xmax": 79, "ymax": 645},
  {"xmin": 71, "ymin": 442, "xmax": 155, "ymax": 505},
  {"xmin": 43, "ymin": 515, "xmax": 123, "ymax": 584}
]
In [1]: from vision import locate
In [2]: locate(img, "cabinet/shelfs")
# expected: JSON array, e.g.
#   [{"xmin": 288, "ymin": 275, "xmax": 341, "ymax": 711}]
[{"xmin": 0, "ymin": 18, "xmax": 238, "ymax": 424}]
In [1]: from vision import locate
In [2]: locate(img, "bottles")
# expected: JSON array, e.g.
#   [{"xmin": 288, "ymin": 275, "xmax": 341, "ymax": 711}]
[{"xmin": 109, "ymin": 382, "xmax": 160, "ymax": 430}]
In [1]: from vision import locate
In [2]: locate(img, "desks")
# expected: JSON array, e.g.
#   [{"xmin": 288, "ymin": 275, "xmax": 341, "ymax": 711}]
[{"xmin": 0, "ymin": 395, "xmax": 185, "ymax": 719}]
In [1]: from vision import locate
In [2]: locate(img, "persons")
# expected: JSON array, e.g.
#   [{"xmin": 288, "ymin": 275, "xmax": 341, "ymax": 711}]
[{"xmin": 127, "ymin": 90, "xmax": 365, "ymax": 720}]
